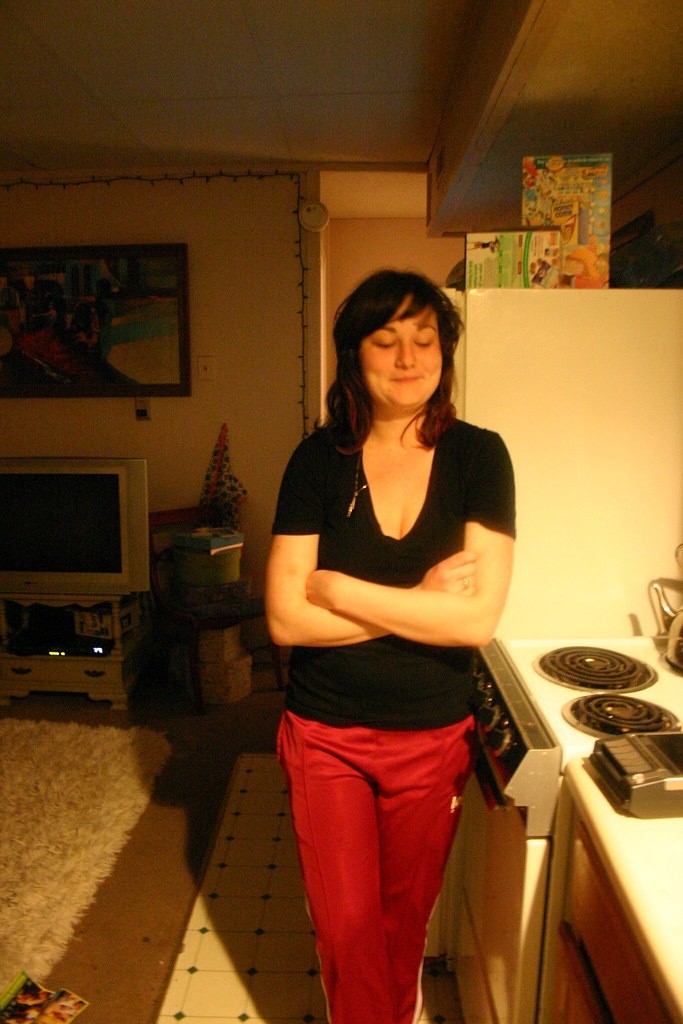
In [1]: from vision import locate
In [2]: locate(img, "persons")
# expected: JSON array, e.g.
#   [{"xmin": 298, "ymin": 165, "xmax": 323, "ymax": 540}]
[{"xmin": 263, "ymin": 269, "xmax": 518, "ymax": 1024}]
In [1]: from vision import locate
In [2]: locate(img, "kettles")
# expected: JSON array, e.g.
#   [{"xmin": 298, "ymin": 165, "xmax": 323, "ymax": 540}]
[{"xmin": 648, "ymin": 579, "xmax": 683, "ymax": 673}]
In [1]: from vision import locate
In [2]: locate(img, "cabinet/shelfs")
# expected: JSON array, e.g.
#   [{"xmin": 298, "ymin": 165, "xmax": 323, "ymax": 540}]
[
  {"xmin": 0, "ymin": 591, "xmax": 154, "ymax": 711},
  {"xmin": 544, "ymin": 840, "xmax": 670, "ymax": 1023}
]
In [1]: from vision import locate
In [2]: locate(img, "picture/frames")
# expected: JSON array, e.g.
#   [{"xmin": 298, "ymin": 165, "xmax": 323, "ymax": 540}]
[{"xmin": 0, "ymin": 241, "xmax": 194, "ymax": 402}]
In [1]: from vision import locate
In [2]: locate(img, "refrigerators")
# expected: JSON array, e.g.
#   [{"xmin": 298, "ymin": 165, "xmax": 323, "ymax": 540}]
[{"xmin": 421, "ymin": 286, "xmax": 683, "ymax": 957}]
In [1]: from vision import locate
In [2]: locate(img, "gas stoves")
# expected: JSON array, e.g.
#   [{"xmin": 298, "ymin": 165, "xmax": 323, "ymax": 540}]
[{"xmin": 475, "ymin": 636, "xmax": 683, "ymax": 837}]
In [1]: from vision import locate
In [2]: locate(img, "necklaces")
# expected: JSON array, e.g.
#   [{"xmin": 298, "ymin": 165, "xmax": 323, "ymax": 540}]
[{"xmin": 346, "ymin": 445, "xmax": 370, "ymax": 519}]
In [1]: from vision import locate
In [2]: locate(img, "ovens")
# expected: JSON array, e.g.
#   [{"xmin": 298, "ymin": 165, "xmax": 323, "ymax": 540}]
[{"xmin": 449, "ymin": 784, "xmax": 573, "ymax": 1024}]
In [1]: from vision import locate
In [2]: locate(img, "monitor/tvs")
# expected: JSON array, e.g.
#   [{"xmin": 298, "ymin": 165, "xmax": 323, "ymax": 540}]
[{"xmin": 0, "ymin": 456, "xmax": 150, "ymax": 595}]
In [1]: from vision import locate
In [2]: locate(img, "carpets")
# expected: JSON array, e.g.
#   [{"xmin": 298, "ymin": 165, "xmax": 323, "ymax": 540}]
[{"xmin": 1, "ymin": 718, "xmax": 176, "ymax": 1003}]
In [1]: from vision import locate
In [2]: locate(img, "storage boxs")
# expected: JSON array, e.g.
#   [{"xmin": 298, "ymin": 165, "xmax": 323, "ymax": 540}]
[
  {"xmin": 164, "ymin": 525, "xmax": 259, "ymax": 705},
  {"xmin": 462, "ymin": 147, "xmax": 620, "ymax": 290}
]
